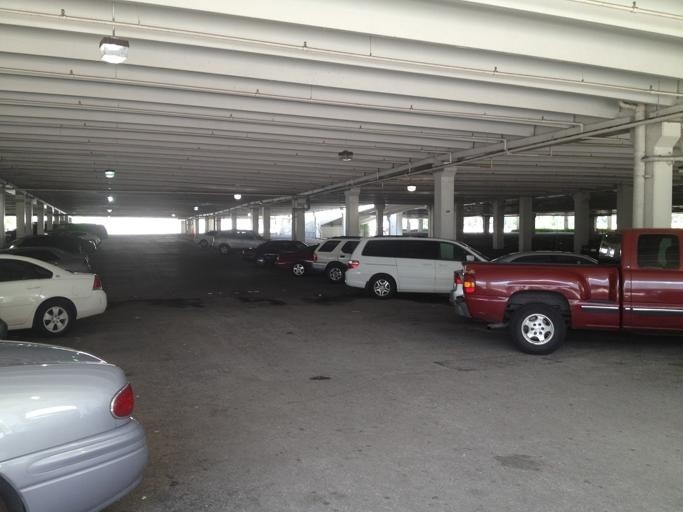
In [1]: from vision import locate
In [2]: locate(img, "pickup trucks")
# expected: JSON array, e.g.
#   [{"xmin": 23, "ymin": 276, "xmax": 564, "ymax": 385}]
[{"xmin": 462, "ymin": 227, "xmax": 683, "ymax": 356}]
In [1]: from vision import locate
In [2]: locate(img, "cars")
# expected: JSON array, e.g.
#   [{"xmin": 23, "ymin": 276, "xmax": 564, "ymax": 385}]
[
  {"xmin": 0, "ymin": 223, "xmax": 109, "ymax": 272},
  {"xmin": 1, "ymin": 340, "xmax": 146, "ymax": 512},
  {"xmin": 1, "ymin": 253, "xmax": 108, "ymax": 340},
  {"xmin": 196, "ymin": 227, "xmax": 598, "ymax": 318}
]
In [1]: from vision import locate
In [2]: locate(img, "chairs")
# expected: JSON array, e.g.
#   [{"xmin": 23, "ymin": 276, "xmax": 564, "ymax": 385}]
[{"xmin": 666, "ymin": 246, "xmax": 680, "ymax": 269}]
